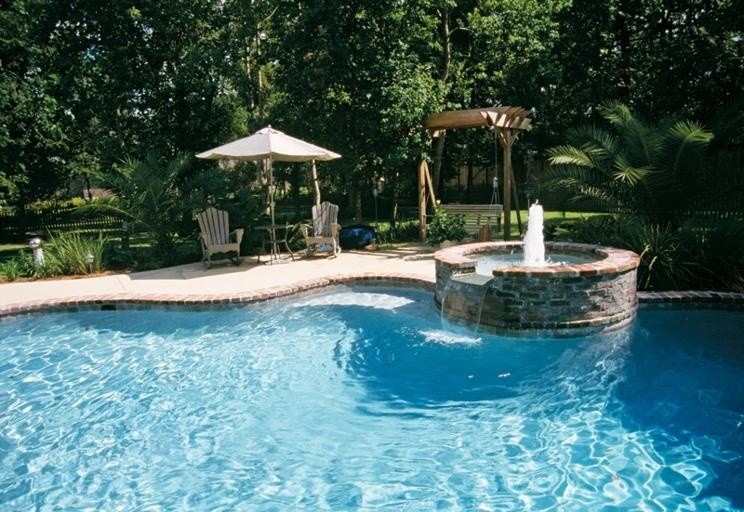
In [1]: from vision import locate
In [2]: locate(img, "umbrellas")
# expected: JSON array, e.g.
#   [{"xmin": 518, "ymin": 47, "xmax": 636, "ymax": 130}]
[{"xmin": 194, "ymin": 124, "xmax": 343, "ymax": 259}]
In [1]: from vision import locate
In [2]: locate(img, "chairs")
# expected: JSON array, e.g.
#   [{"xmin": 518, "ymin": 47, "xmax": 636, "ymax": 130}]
[
  {"xmin": 196, "ymin": 207, "xmax": 245, "ymax": 270},
  {"xmin": 300, "ymin": 200, "xmax": 341, "ymax": 258}
]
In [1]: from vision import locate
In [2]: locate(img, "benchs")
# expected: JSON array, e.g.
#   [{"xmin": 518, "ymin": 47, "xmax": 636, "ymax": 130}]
[{"xmin": 440, "ymin": 203, "xmax": 502, "ymax": 232}]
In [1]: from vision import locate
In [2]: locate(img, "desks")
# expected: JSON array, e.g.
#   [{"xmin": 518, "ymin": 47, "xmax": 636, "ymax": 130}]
[{"xmin": 255, "ymin": 225, "xmax": 295, "ymax": 262}]
